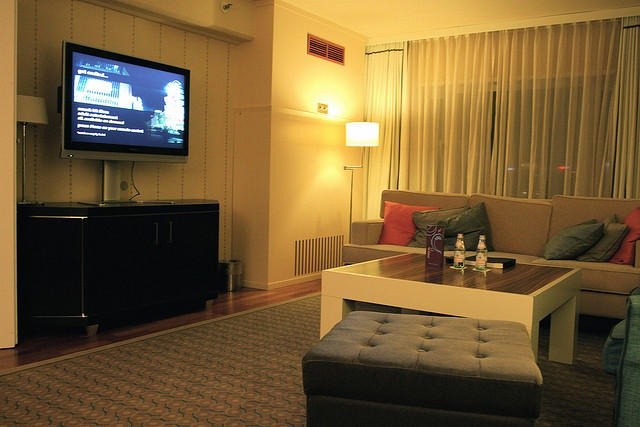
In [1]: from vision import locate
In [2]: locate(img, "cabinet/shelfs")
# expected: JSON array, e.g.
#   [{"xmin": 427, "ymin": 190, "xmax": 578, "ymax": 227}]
[{"xmin": 17, "ymin": 199, "xmax": 220, "ymax": 336}]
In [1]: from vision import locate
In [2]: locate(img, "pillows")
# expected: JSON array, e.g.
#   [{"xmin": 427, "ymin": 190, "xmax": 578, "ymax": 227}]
[
  {"xmin": 543, "ymin": 219, "xmax": 605, "ymax": 260},
  {"xmin": 377, "ymin": 200, "xmax": 441, "ymax": 246},
  {"xmin": 468, "ymin": 193, "xmax": 553, "ymax": 256},
  {"xmin": 437, "ymin": 201, "xmax": 495, "ymax": 251},
  {"xmin": 608, "ymin": 207, "xmax": 640, "ymax": 265},
  {"xmin": 575, "ymin": 214, "xmax": 630, "ymax": 262},
  {"xmin": 380, "ymin": 190, "xmax": 468, "ymax": 218},
  {"xmin": 406, "ymin": 206, "xmax": 470, "ymax": 248},
  {"xmin": 548, "ymin": 195, "xmax": 640, "ymax": 241}
]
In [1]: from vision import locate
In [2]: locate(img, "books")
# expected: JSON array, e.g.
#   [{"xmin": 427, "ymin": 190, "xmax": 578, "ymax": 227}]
[{"xmin": 465, "ymin": 256, "xmax": 516, "ymax": 269}]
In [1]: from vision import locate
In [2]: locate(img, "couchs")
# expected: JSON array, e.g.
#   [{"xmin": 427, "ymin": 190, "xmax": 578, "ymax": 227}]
[
  {"xmin": 342, "ymin": 190, "xmax": 640, "ymax": 319},
  {"xmin": 302, "ymin": 311, "xmax": 544, "ymax": 427},
  {"xmin": 603, "ymin": 286, "xmax": 639, "ymax": 427}
]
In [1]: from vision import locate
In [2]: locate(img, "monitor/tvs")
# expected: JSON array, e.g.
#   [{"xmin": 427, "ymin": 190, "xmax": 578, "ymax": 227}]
[{"xmin": 57, "ymin": 40, "xmax": 191, "ymax": 205}]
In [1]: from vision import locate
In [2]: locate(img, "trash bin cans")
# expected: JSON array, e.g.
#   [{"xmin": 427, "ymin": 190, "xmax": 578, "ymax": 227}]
[{"xmin": 219, "ymin": 260, "xmax": 242, "ymax": 292}]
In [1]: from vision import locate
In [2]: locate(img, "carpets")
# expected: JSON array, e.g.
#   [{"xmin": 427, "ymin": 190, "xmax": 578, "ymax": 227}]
[{"xmin": 1, "ymin": 291, "xmax": 616, "ymax": 427}]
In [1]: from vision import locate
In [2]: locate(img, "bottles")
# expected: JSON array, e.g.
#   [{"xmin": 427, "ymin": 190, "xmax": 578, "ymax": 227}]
[
  {"xmin": 453, "ymin": 233, "xmax": 467, "ymax": 273},
  {"xmin": 475, "ymin": 233, "xmax": 489, "ymax": 274}
]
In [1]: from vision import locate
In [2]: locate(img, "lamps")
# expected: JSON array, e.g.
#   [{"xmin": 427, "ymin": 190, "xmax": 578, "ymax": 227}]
[
  {"xmin": 344, "ymin": 122, "xmax": 380, "ymax": 243},
  {"xmin": 17, "ymin": 94, "xmax": 49, "ymax": 204}
]
